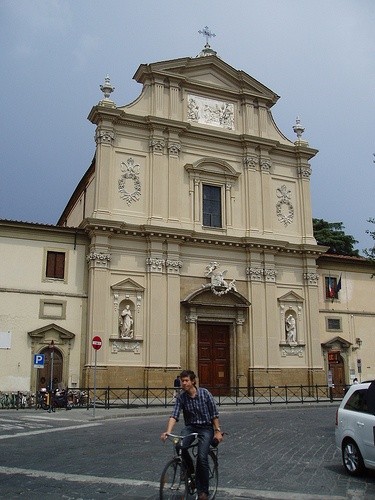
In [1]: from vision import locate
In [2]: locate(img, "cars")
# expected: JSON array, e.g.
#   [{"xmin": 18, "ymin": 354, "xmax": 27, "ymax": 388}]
[{"xmin": 335, "ymin": 380, "xmax": 375, "ymax": 474}]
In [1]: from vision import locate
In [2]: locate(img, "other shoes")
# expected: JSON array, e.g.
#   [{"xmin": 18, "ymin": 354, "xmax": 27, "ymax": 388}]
[{"xmin": 198, "ymin": 492, "xmax": 208, "ymax": 499}]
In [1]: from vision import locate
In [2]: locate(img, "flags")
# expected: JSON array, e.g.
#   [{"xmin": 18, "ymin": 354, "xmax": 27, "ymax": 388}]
[
  {"xmin": 328, "ymin": 275, "xmax": 335, "ymax": 298},
  {"xmin": 334, "ymin": 276, "xmax": 341, "ymax": 293}
]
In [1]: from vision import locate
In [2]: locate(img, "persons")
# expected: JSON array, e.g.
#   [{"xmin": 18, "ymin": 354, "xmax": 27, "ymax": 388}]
[
  {"xmin": 353, "ymin": 377, "xmax": 358, "ymax": 384},
  {"xmin": 160, "ymin": 369, "xmax": 223, "ymax": 500},
  {"xmin": 173, "ymin": 375, "xmax": 181, "ymax": 395},
  {"xmin": 120, "ymin": 305, "xmax": 132, "ymax": 337},
  {"xmin": 38, "ymin": 376, "xmax": 58, "ymax": 388},
  {"xmin": 285, "ymin": 314, "xmax": 296, "ymax": 342}
]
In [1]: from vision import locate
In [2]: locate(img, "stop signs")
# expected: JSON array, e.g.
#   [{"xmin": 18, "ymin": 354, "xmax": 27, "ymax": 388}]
[{"xmin": 92, "ymin": 336, "xmax": 102, "ymax": 350}]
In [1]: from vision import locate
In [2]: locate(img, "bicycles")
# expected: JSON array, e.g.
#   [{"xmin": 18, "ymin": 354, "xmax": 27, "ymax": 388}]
[
  {"xmin": 0, "ymin": 388, "xmax": 91, "ymax": 412},
  {"xmin": 159, "ymin": 431, "xmax": 220, "ymax": 499}
]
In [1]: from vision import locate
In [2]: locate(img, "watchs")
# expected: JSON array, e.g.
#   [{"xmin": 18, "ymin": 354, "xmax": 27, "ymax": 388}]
[{"xmin": 215, "ymin": 427, "xmax": 220, "ymax": 432}]
